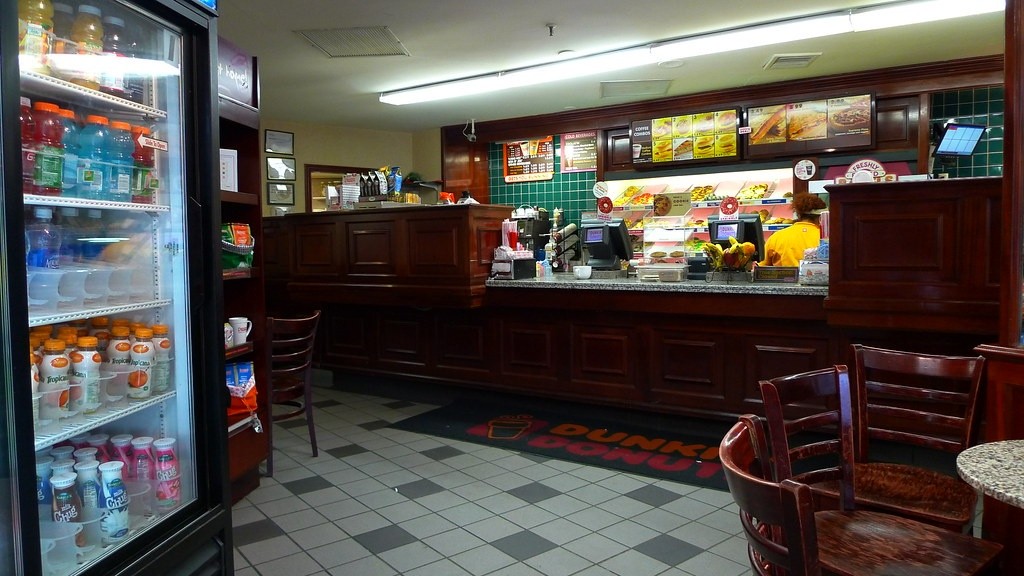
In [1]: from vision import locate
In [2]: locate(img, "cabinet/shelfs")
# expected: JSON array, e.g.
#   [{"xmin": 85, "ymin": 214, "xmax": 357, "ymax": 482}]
[{"xmin": 217, "ymin": 91, "xmax": 273, "ymax": 476}]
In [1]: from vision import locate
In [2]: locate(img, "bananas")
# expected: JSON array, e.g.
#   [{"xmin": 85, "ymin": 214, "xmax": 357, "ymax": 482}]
[{"xmin": 703, "ymin": 235, "xmax": 745, "ymax": 268}]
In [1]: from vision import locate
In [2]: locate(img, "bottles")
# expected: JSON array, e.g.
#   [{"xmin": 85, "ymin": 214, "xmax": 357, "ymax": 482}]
[{"xmin": 16, "ymin": 0, "xmax": 182, "ymax": 545}]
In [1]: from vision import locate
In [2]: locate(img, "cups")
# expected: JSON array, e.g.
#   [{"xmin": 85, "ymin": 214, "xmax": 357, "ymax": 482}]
[
  {"xmin": 229, "ymin": 317, "xmax": 252, "ymax": 346},
  {"xmin": 529, "ymin": 139, "xmax": 539, "ymax": 154},
  {"xmin": 520, "ymin": 142, "xmax": 529, "ymax": 156},
  {"xmin": 633, "ymin": 144, "xmax": 642, "ymax": 158},
  {"xmin": 573, "ymin": 266, "xmax": 592, "ymax": 279}
]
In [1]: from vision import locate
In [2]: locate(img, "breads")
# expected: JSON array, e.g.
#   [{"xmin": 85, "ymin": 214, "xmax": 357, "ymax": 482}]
[
  {"xmin": 612, "ymin": 186, "xmax": 654, "ymax": 229},
  {"xmin": 685, "ymin": 237, "xmax": 708, "ymax": 252},
  {"xmin": 687, "ymin": 183, "xmax": 795, "ymax": 224},
  {"xmin": 653, "ymin": 195, "xmax": 672, "ymax": 216},
  {"xmin": 751, "ymin": 106, "xmax": 786, "ymax": 142}
]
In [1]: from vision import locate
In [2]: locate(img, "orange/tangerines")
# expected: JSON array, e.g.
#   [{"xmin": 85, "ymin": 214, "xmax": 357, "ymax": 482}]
[{"xmin": 742, "ymin": 242, "xmax": 755, "ymax": 255}]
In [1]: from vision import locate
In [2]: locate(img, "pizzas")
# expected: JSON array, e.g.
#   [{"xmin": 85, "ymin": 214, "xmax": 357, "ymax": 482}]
[
  {"xmin": 830, "ymin": 105, "xmax": 872, "ymax": 131},
  {"xmin": 787, "ymin": 112, "xmax": 826, "ymax": 138}
]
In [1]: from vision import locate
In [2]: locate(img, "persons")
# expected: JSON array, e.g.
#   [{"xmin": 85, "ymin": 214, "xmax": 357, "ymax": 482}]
[{"xmin": 754, "ymin": 188, "xmax": 827, "ymax": 266}]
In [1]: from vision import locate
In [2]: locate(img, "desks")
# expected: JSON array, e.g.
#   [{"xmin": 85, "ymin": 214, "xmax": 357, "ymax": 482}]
[{"xmin": 956, "ymin": 440, "xmax": 1024, "ymax": 539}]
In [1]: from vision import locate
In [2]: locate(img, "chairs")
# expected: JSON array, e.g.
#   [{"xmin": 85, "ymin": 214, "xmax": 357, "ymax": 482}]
[
  {"xmin": 718, "ymin": 343, "xmax": 1003, "ymax": 576},
  {"xmin": 263, "ymin": 310, "xmax": 323, "ymax": 477}
]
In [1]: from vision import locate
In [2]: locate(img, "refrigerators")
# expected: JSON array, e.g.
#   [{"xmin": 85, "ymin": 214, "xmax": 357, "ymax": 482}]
[{"xmin": 0, "ymin": 1, "xmax": 237, "ymax": 576}]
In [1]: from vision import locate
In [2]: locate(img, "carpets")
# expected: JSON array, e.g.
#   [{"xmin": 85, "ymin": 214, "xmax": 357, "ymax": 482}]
[{"xmin": 384, "ymin": 400, "xmax": 949, "ymax": 492}]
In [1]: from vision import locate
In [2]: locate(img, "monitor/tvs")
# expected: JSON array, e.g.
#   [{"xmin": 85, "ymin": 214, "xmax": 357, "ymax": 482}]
[
  {"xmin": 581, "ymin": 217, "xmax": 634, "ymax": 261},
  {"xmin": 707, "ymin": 214, "xmax": 765, "ymax": 263},
  {"xmin": 931, "ymin": 123, "xmax": 986, "ymax": 159}
]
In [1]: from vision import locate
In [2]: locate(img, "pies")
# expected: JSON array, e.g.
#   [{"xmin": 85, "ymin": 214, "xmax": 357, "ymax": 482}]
[{"xmin": 654, "ymin": 112, "xmax": 737, "ymax": 158}]
